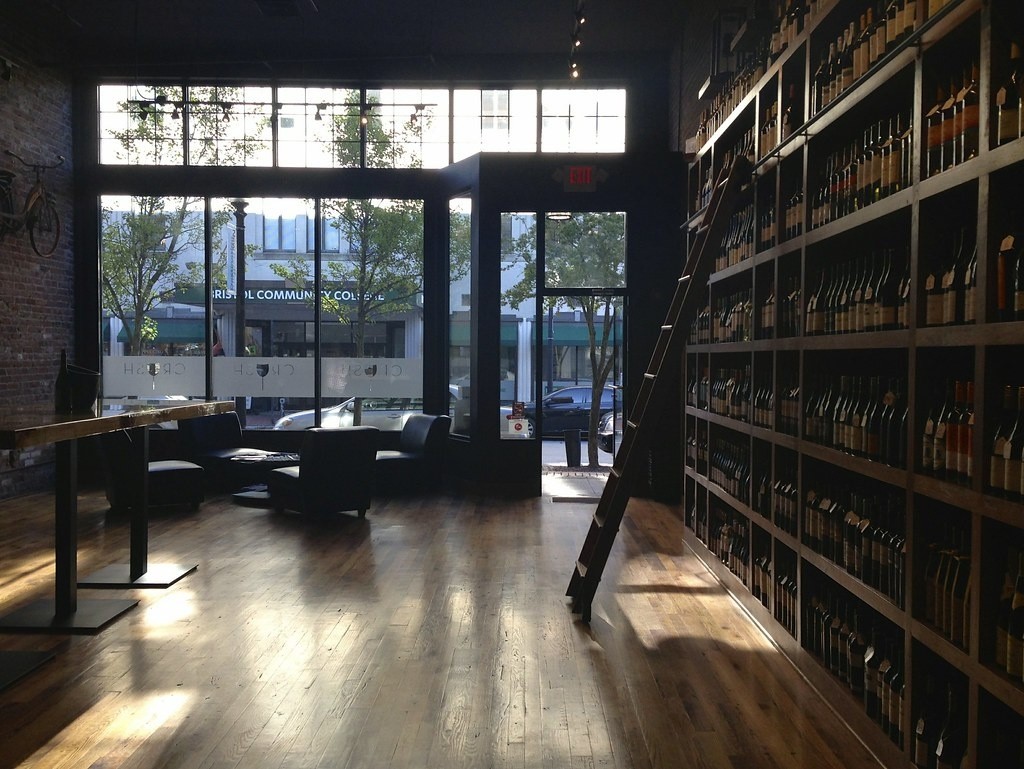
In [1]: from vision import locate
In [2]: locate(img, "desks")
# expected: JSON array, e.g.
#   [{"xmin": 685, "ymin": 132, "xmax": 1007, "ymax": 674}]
[{"xmin": 0, "ymin": 397, "xmax": 235, "ymax": 636}]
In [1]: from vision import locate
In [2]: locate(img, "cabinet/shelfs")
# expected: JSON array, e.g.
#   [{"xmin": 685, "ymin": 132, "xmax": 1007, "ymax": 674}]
[{"xmin": 684, "ymin": 1, "xmax": 1024, "ymax": 769}]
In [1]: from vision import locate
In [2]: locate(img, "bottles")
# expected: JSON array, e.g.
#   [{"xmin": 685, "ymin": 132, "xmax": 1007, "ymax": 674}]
[{"xmin": 685, "ymin": 0, "xmax": 1024, "ymax": 767}]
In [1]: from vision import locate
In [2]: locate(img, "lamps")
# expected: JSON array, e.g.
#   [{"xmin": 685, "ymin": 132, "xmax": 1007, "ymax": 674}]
[
  {"xmin": 360, "ymin": 105, "xmax": 372, "ymax": 124},
  {"xmin": 567, "ymin": 54, "xmax": 578, "ymax": 78},
  {"xmin": 171, "ymin": 103, "xmax": 184, "ymax": 119},
  {"xmin": 569, "ymin": 33, "xmax": 581, "ymax": 47},
  {"xmin": 411, "ymin": 104, "xmax": 425, "ymax": 123},
  {"xmin": 139, "ymin": 101, "xmax": 149, "ymax": 121},
  {"xmin": 270, "ymin": 103, "xmax": 282, "ymax": 121},
  {"xmin": 221, "ymin": 103, "xmax": 231, "ymax": 122},
  {"xmin": 572, "ymin": 10, "xmax": 586, "ymax": 24},
  {"xmin": 315, "ymin": 105, "xmax": 327, "ymax": 119}
]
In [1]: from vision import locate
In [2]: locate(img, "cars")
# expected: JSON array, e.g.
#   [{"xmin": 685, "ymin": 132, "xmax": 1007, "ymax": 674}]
[
  {"xmin": 596, "ymin": 408, "xmax": 624, "ymax": 454},
  {"xmin": 272, "ymin": 382, "xmax": 514, "ymax": 453},
  {"xmin": 510, "ymin": 383, "xmax": 623, "ymax": 439}
]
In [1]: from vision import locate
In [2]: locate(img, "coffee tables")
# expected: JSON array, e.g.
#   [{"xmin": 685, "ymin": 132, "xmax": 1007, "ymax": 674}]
[{"xmin": 230, "ymin": 453, "xmax": 301, "ymax": 508}]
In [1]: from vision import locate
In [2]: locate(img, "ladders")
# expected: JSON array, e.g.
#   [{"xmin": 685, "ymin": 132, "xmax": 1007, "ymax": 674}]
[{"xmin": 564, "ymin": 156, "xmax": 753, "ymax": 624}]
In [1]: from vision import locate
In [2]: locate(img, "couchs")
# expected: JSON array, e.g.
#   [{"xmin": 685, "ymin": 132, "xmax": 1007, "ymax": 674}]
[
  {"xmin": 372, "ymin": 414, "xmax": 452, "ymax": 499},
  {"xmin": 266, "ymin": 425, "xmax": 380, "ymax": 523},
  {"xmin": 177, "ymin": 411, "xmax": 268, "ymax": 496},
  {"xmin": 91, "ymin": 426, "xmax": 205, "ymax": 516}
]
